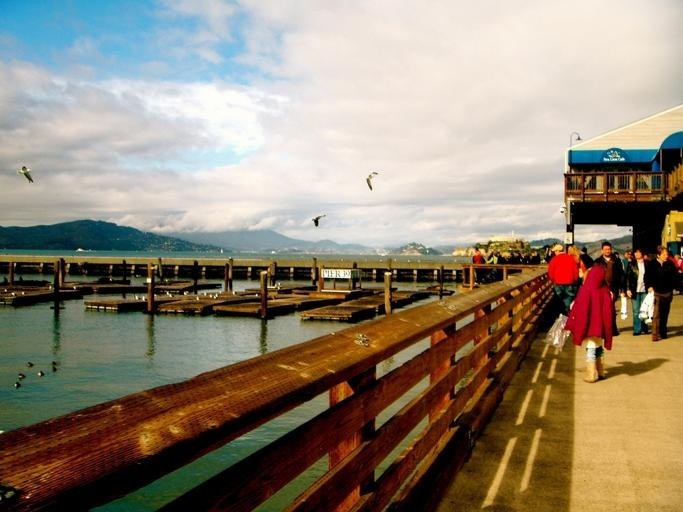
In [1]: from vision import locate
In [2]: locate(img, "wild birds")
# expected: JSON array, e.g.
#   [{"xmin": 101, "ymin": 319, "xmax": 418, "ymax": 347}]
[
  {"xmin": 135, "ymin": 290, "xmax": 237, "ymax": 301},
  {"xmin": 312, "ymin": 214, "xmax": 326, "ymax": 227},
  {"xmin": 14, "ymin": 361, "xmax": 33, "ymax": 388},
  {"xmin": 367, "ymin": 172, "xmax": 378, "ymax": 191},
  {"xmin": 4, "ymin": 287, "xmax": 25, "ymax": 296},
  {"xmin": 18, "ymin": 166, "xmax": 34, "ymax": 184},
  {"xmin": 37, "ymin": 360, "xmax": 57, "ymax": 376}
]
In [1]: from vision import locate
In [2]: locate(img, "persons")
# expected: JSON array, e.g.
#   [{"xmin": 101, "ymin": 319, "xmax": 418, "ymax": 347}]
[
  {"xmin": 564, "ymin": 265, "xmax": 614, "ymax": 383},
  {"xmin": 473, "ymin": 242, "xmax": 683, "ymax": 341}
]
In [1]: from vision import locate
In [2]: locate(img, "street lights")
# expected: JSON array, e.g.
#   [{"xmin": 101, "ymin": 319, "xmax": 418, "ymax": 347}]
[{"xmin": 569, "ymin": 131, "xmax": 581, "ymax": 147}]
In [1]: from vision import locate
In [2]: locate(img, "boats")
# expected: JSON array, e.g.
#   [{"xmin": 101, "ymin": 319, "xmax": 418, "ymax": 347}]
[{"xmin": 74, "ymin": 248, "xmax": 96, "ymax": 254}]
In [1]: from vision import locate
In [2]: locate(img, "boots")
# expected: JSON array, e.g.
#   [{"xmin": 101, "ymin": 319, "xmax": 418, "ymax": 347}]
[{"xmin": 583, "ymin": 356, "xmax": 607, "ymax": 382}]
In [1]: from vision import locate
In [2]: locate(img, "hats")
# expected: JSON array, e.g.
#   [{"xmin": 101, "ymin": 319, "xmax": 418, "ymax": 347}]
[{"xmin": 551, "ymin": 244, "xmax": 563, "ymax": 251}]
